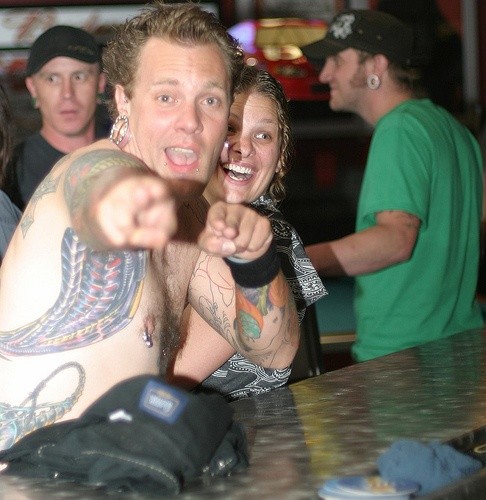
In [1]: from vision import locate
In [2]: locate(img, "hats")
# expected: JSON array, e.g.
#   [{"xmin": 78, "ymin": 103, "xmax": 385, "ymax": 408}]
[
  {"xmin": 298, "ymin": 8, "xmax": 417, "ymax": 67},
  {"xmin": 23, "ymin": 25, "xmax": 101, "ymax": 77}
]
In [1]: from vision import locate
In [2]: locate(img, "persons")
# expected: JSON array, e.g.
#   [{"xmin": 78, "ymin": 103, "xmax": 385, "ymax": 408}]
[
  {"xmin": 176, "ymin": 63, "xmax": 328, "ymax": 403},
  {"xmin": 0, "ymin": 77, "xmax": 24, "ymax": 264},
  {"xmin": 1, "ymin": 25, "xmax": 114, "ymax": 214},
  {"xmin": 0, "ymin": 0, "xmax": 248, "ymax": 472},
  {"xmin": 298, "ymin": 9, "xmax": 484, "ymax": 363}
]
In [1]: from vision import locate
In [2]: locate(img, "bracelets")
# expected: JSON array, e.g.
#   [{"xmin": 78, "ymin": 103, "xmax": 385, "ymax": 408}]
[{"xmin": 223, "ymin": 240, "xmax": 281, "ymax": 289}]
